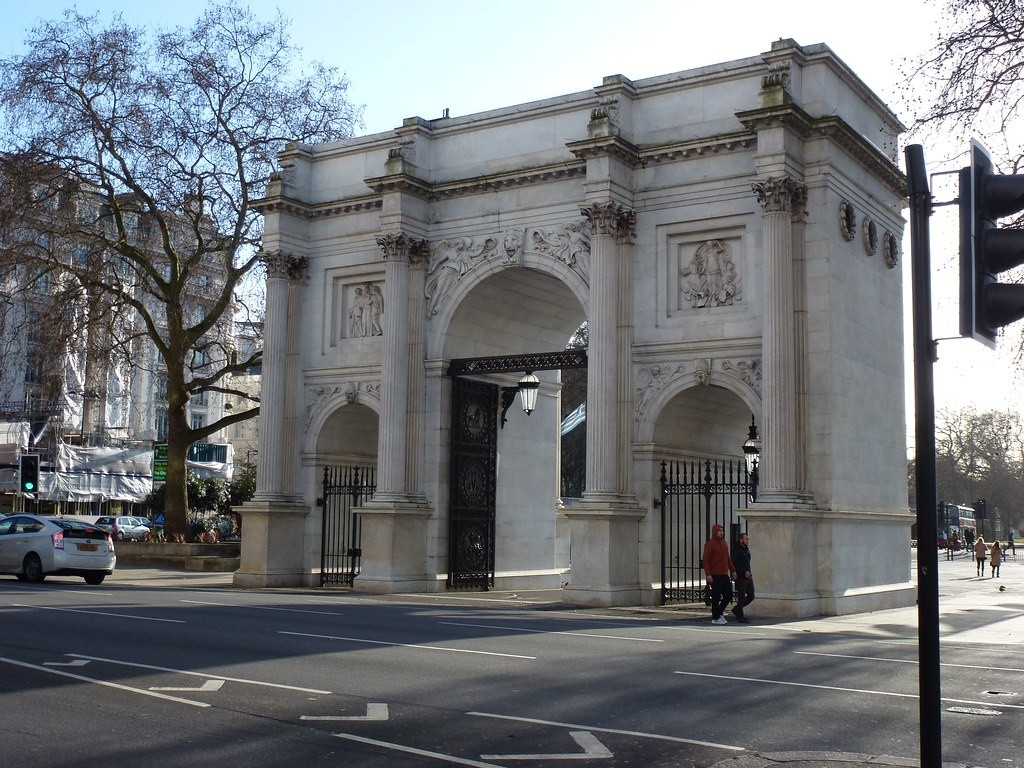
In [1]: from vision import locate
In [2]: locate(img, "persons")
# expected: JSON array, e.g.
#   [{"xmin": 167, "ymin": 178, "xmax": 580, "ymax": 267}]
[
  {"xmin": 963, "ymin": 527, "xmax": 975, "ymax": 553},
  {"xmin": 635, "ymin": 365, "xmax": 670, "ymax": 421},
  {"xmin": 738, "ymin": 359, "xmax": 762, "ymax": 394},
  {"xmin": 991, "ymin": 540, "xmax": 1002, "ymax": 578},
  {"xmin": 303, "ymin": 387, "xmax": 327, "ymax": 433},
  {"xmin": 953, "ymin": 530, "xmax": 959, "ymax": 551},
  {"xmin": 349, "ymin": 286, "xmax": 384, "ymax": 338},
  {"xmin": 974, "ymin": 537, "xmax": 989, "ymax": 576},
  {"xmin": 699, "ymin": 239, "xmax": 725, "ymax": 295},
  {"xmin": 703, "ymin": 525, "xmax": 755, "ymax": 625},
  {"xmin": 424, "ymin": 238, "xmax": 486, "ymax": 318},
  {"xmin": 1003, "ymin": 529, "xmax": 1017, "ymax": 555},
  {"xmin": 533, "ymin": 223, "xmax": 591, "ymax": 290}
]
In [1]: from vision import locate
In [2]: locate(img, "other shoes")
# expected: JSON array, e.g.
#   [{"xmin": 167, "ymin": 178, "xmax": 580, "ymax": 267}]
[
  {"xmin": 712, "ymin": 615, "xmax": 728, "ymax": 624},
  {"xmin": 732, "ymin": 607, "xmax": 749, "ymax": 623},
  {"xmin": 997, "ymin": 576, "xmax": 999, "ymax": 577}
]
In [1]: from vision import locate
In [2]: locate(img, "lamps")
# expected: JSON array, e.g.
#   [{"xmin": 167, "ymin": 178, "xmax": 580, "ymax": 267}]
[
  {"xmin": 501, "ymin": 371, "xmax": 541, "ymax": 430},
  {"xmin": 742, "ymin": 413, "xmax": 760, "ymax": 477}
]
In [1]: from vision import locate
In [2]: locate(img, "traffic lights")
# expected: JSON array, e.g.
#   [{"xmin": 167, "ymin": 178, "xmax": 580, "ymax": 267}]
[
  {"xmin": 18, "ymin": 453, "xmax": 41, "ymax": 497},
  {"xmin": 968, "ymin": 135, "xmax": 1024, "ymax": 351}
]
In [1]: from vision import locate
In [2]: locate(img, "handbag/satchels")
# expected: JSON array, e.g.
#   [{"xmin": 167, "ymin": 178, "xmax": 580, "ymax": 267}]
[{"xmin": 990, "ymin": 561, "xmax": 992, "ymax": 566}]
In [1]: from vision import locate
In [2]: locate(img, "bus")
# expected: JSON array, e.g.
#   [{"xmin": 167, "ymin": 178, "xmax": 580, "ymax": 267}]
[{"xmin": 944, "ymin": 503, "xmax": 977, "ymax": 538}]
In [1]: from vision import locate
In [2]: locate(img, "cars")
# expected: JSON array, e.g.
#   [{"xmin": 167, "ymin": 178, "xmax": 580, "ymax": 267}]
[
  {"xmin": 94, "ymin": 516, "xmax": 151, "ymax": 542},
  {"xmin": 0, "ymin": 513, "xmax": 24, "ymax": 533},
  {"xmin": 133, "ymin": 517, "xmax": 151, "ymax": 526},
  {"xmin": 0, "ymin": 514, "xmax": 117, "ymax": 584}
]
